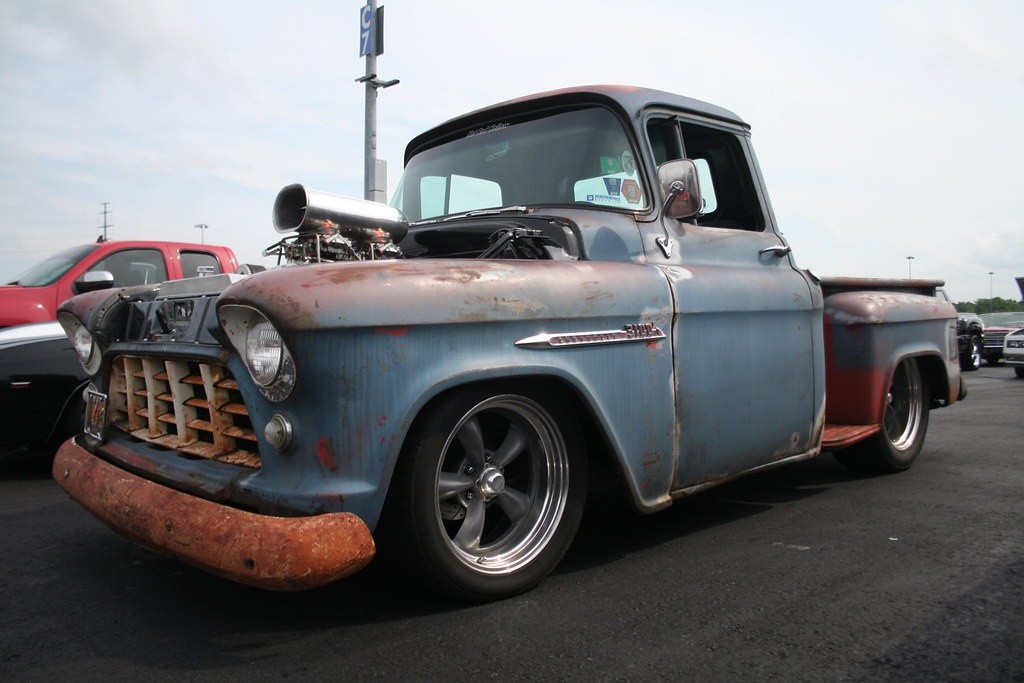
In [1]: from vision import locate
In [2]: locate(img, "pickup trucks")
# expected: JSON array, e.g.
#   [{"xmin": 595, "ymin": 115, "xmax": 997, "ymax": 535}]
[
  {"xmin": 47, "ymin": 81, "xmax": 973, "ymax": 601},
  {"xmin": 933, "ymin": 287, "xmax": 987, "ymax": 370}
]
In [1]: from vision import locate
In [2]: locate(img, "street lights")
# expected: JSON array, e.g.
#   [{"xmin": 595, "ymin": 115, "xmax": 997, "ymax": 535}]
[
  {"xmin": 906, "ymin": 254, "xmax": 916, "ymax": 279},
  {"xmin": 194, "ymin": 224, "xmax": 210, "ymax": 246},
  {"xmin": 988, "ymin": 268, "xmax": 996, "ymax": 316}
]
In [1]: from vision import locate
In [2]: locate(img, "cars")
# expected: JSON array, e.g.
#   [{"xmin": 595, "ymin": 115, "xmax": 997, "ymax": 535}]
[
  {"xmin": 0, "ymin": 320, "xmax": 89, "ymax": 470},
  {"xmin": 0, "ymin": 239, "xmax": 240, "ymax": 333},
  {"xmin": 971, "ymin": 309, "xmax": 1024, "ymax": 364},
  {"xmin": 1003, "ymin": 328, "xmax": 1024, "ymax": 379}
]
